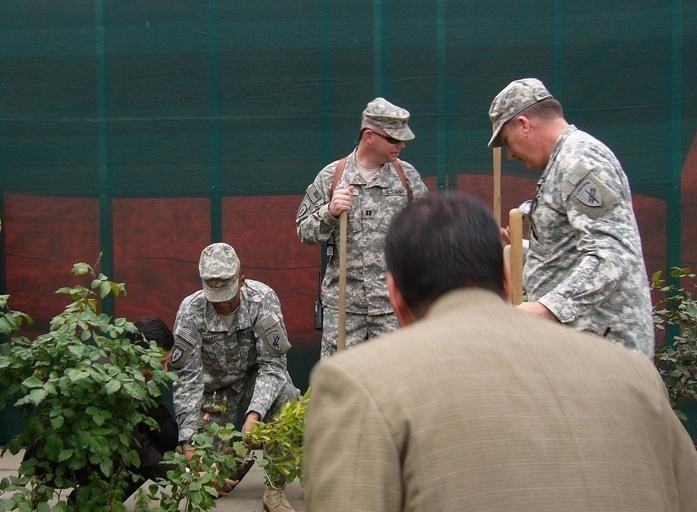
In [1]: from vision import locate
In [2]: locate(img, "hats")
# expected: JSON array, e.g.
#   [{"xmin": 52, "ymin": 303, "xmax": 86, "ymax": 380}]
[
  {"xmin": 198, "ymin": 243, "xmax": 240, "ymax": 303},
  {"xmin": 363, "ymin": 98, "xmax": 415, "ymax": 141},
  {"xmin": 488, "ymin": 78, "xmax": 552, "ymax": 148}
]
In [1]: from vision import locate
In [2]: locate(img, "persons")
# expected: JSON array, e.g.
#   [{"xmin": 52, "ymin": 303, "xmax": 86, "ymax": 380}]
[
  {"xmin": 170, "ymin": 242, "xmax": 301, "ymax": 512},
  {"xmin": 486, "ymin": 77, "xmax": 656, "ymax": 364},
  {"xmin": 295, "ymin": 96, "xmax": 431, "ymax": 360},
  {"xmin": 303, "ymin": 194, "xmax": 697, "ymax": 512},
  {"xmin": 21, "ymin": 316, "xmax": 178, "ymax": 512}
]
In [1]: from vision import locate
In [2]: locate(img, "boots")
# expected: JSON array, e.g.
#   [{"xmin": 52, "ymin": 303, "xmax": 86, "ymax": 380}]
[
  {"xmin": 214, "ymin": 448, "xmax": 257, "ymax": 499},
  {"xmin": 263, "ymin": 481, "xmax": 296, "ymax": 512}
]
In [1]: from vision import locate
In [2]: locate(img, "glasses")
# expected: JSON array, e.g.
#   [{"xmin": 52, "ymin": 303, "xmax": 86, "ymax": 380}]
[{"xmin": 374, "ymin": 132, "xmax": 400, "ymax": 144}]
